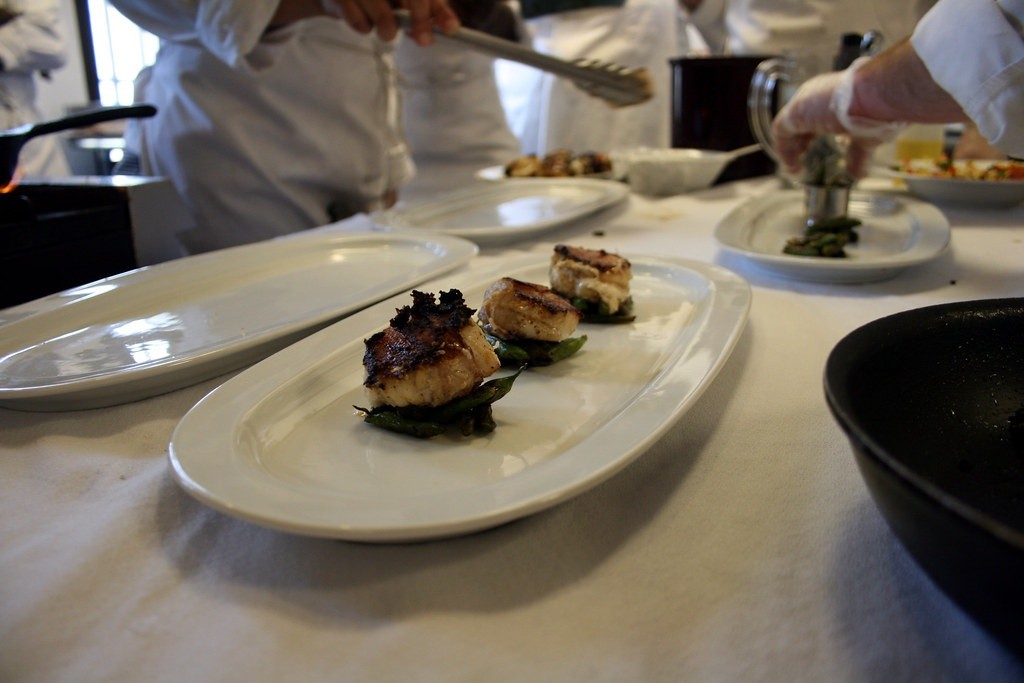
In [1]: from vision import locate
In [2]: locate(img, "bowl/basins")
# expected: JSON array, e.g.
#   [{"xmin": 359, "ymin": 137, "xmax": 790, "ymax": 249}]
[
  {"xmin": 824, "ymin": 295, "xmax": 1024, "ymax": 664},
  {"xmin": 610, "ymin": 145, "xmax": 734, "ymax": 198}
]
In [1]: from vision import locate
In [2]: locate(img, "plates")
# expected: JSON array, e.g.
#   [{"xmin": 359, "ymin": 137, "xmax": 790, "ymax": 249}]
[
  {"xmin": 713, "ymin": 188, "xmax": 949, "ymax": 284},
  {"xmin": 167, "ymin": 247, "xmax": 751, "ymax": 541},
  {"xmin": 474, "ymin": 163, "xmax": 625, "ymax": 182},
  {"xmin": 22, "ymin": 228, "xmax": 476, "ymax": 410},
  {"xmin": 882, "ymin": 159, "xmax": 1024, "ymax": 207},
  {"xmin": 384, "ymin": 175, "xmax": 631, "ymax": 242}
]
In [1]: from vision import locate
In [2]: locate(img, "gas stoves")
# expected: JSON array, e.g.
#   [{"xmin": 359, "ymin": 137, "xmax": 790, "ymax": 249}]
[{"xmin": 0, "ymin": 173, "xmax": 179, "ymax": 308}]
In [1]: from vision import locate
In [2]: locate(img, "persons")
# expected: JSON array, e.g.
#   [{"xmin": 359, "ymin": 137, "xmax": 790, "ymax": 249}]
[
  {"xmin": 104, "ymin": 0, "xmax": 524, "ymax": 271},
  {"xmin": 0, "ymin": 0, "xmax": 72, "ymax": 180},
  {"xmin": 769, "ymin": 0, "xmax": 1023, "ymax": 188},
  {"xmin": 390, "ymin": 0, "xmax": 1023, "ymax": 198}
]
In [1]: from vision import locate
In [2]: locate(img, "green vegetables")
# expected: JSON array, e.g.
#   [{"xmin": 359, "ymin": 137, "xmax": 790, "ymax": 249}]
[{"xmin": 351, "ymin": 293, "xmax": 637, "ymax": 439}]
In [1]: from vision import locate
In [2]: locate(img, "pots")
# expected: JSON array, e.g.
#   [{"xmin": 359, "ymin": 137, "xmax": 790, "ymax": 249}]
[{"xmin": 0, "ymin": 104, "xmax": 156, "ymax": 191}]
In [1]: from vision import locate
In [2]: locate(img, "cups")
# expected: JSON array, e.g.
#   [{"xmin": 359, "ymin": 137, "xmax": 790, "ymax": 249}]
[
  {"xmin": 668, "ymin": 55, "xmax": 778, "ymax": 176},
  {"xmin": 893, "ymin": 123, "xmax": 943, "ymax": 187}
]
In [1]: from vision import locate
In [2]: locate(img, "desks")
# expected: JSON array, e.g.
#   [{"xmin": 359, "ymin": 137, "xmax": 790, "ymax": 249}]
[{"xmin": 0, "ymin": 175, "xmax": 1024, "ymax": 683}]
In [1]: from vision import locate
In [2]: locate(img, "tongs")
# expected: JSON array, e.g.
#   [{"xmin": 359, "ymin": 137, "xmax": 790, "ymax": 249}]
[{"xmin": 394, "ymin": 9, "xmax": 655, "ymax": 106}]
